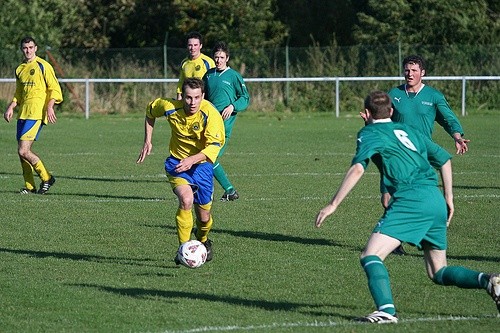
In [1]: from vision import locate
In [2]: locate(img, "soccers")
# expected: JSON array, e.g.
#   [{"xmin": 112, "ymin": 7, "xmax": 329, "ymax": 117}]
[{"xmin": 177, "ymin": 239, "xmax": 208, "ymax": 268}]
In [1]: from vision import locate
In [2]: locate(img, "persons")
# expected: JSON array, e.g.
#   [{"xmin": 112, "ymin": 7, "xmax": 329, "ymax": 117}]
[
  {"xmin": 359, "ymin": 56, "xmax": 472, "ymax": 255},
  {"xmin": 136, "ymin": 78, "xmax": 226, "ymax": 265},
  {"xmin": 315, "ymin": 90, "xmax": 500, "ymax": 325},
  {"xmin": 174, "ymin": 32, "xmax": 217, "ymax": 101},
  {"xmin": 201, "ymin": 44, "xmax": 251, "ymax": 203},
  {"xmin": 4, "ymin": 36, "xmax": 64, "ymax": 195}
]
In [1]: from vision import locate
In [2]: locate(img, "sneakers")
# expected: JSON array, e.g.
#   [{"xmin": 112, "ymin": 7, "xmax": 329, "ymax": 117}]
[
  {"xmin": 220, "ymin": 191, "xmax": 239, "ymax": 201},
  {"xmin": 356, "ymin": 310, "xmax": 399, "ymax": 325},
  {"xmin": 37, "ymin": 175, "xmax": 56, "ymax": 194},
  {"xmin": 487, "ymin": 274, "xmax": 500, "ymax": 312},
  {"xmin": 19, "ymin": 188, "xmax": 37, "ymax": 195},
  {"xmin": 174, "ymin": 254, "xmax": 180, "ymax": 265},
  {"xmin": 203, "ymin": 240, "xmax": 214, "ymax": 261}
]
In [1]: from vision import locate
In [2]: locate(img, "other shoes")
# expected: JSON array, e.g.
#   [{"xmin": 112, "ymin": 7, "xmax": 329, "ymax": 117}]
[{"xmin": 394, "ymin": 246, "xmax": 407, "ymax": 255}]
